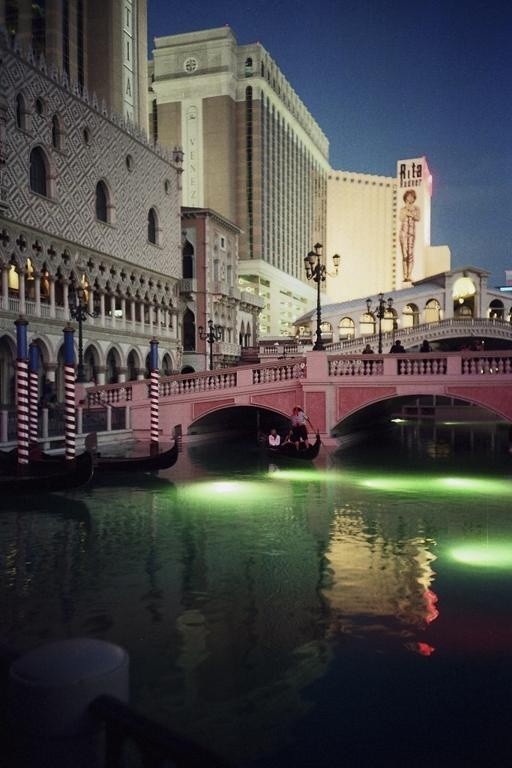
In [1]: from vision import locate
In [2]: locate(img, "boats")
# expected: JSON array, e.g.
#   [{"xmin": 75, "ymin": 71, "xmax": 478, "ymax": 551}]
[
  {"xmin": 255, "ymin": 426, "xmax": 321, "ymax": 461},
  {"xmin": 8, "ymin": 424, "xmax": 183, "ymax": 476},
  {"xmin": 0, "ymin": 432, "xmax": 99, "ymax": 496}
]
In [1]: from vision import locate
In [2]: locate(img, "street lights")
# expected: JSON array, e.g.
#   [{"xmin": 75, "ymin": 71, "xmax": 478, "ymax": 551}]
[
  {"xmin": 365, "ymin": 292, "xmax": 394, "ymax": 354},
  {"xmin": 303, "ymin": 242, "xmax": 340, "ymax": 351},
  {"xmin": 68, "ymin": 285, "xmax": 100, "ymax": 383},
  {"xmin": 198, "ymin": 319, "xmax": 224, "ymax": 371}
]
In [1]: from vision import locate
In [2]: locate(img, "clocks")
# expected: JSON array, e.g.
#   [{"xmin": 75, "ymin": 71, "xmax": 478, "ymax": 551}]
[{"xmin": 183, "ymin": 56, "xmax": 197, "ymax": 73}]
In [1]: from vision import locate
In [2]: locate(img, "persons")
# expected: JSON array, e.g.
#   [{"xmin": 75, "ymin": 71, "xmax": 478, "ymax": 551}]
[
  {"xmin": 420, "ymin": 340, "xmax": 433, "ymax": 351},
  {"xmin": 268, "ymin": 428, "xmax": 280, "ymax": 446},
  {"xmin": 398, "ymin": 188, "xmax": 420, "ymax": 282},
  {"xmin": 281, "ymin": 429, "xmax": 302, "ymax": 445},
  {"xmin": 288, "ymin": 405, "xmax": 312, "ymax": 450},
  {"xmin": 390, "ymin": 340, "xmax": 406, "ymax": 353},
  {"xmin": 362, "ymin": 343, "xmax": 375, "ymax": 353}
]
